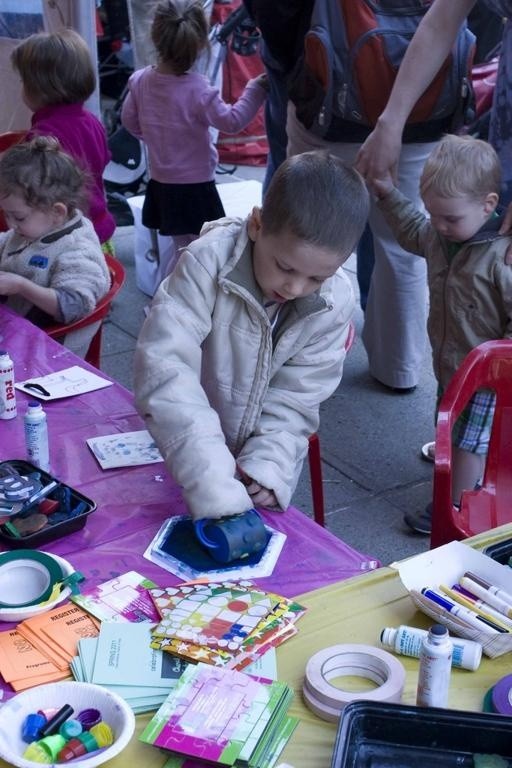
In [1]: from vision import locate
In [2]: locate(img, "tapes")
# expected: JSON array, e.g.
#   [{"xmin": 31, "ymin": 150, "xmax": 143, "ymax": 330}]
[
  {"xmin": 0, "ymin": 549, "xmax": 64, "ymax": 608},
  {"xmin": 492, "ymin": 673, "xmax": 512, "ymax": 716},
  {"xmin": 303, "ymin": 643, "xmax": 405, "ymax": 724}
]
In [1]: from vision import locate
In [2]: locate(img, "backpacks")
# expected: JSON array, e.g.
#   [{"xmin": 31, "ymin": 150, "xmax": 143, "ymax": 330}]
[{"xmin": 296, "ymin": 1, "xmax": 477, "ymax": 125}]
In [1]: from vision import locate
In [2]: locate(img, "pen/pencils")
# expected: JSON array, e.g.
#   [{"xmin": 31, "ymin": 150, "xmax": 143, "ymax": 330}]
[{"xmin": 419, "ymin": 570, "xmax": 511, "ymax": 633}]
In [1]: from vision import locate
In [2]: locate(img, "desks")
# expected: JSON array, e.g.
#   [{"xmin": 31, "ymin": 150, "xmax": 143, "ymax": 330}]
[
  {"xmin": 0, "ymin": 303, "xmax": 382, "ymax": 703},
  {"xmin": 0, "ymin": 523, "xmax": 512, "ymax": 768}
]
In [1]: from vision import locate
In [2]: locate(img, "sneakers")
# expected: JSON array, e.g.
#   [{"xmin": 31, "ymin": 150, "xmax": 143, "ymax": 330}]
[{"xmin": 404, "ymin": 498, "xmax": 461, "ymax": 533}]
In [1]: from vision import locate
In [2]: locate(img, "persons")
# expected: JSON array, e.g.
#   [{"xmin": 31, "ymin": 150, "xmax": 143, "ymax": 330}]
[
  {"xmin": 0, "ymin": 0, "xmax": 267, "ymax": 362},
  {"xmin": 244, "ymin": 0, "xmax": 511, "ymax": 537},
  {"xmin": 139, "ymin": 150, "xmax": 370, "ymax": 519}
]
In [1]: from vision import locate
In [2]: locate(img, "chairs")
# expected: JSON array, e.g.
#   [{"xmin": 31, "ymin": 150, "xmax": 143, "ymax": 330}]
[
  {"xmin": 308, "ymin": 319, "xmax": 355, "ymax": 528},
  {"xmin": 42, "ymin": 253, "xmax": 126, "ymax": 369},
  {"xmin": 0, "ymin": 129, "xmax": 26, "ymax": 232},
  {"xmin": 431, "ymin": 339, "xmax": 512, "ymax": 549}
]
